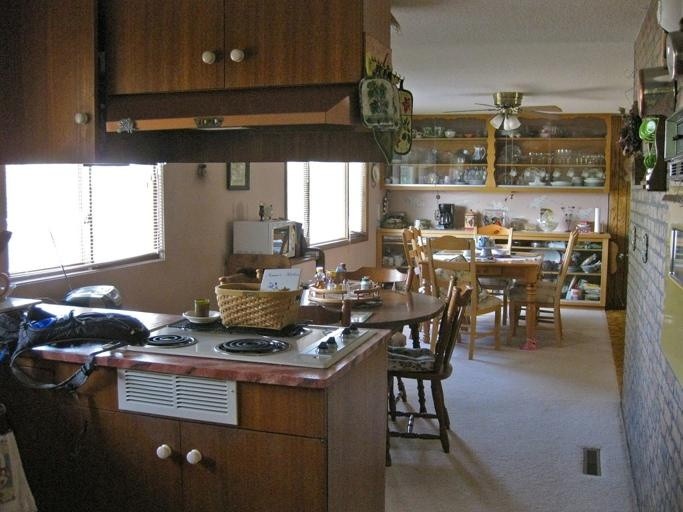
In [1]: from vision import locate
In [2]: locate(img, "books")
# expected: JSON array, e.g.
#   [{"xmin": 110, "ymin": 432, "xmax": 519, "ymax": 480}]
[
  {"xmin": 260, "ymin": 268, "xmax": 301, "ymax": 291},
  {"xmin": 351, "ymin": 311, "xmax": 373, "ymax": 324}
]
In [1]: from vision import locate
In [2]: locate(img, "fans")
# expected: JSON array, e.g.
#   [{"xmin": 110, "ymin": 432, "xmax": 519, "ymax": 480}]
[{"xmin": 444, "ymin": 91, "xmax": 562, "ymax": 120}]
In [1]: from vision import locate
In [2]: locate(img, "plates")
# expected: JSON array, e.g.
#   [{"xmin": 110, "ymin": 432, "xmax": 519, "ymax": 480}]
[
  {"xmin": 522, "ymin": 167, "xmax": 539, "ymax": 184},
  {"xmin": 468, "ymin": 179, "xmax": 483, "ymax": 185},
  {"xmin": 527, "ymin": 177, "xmax": 604, "ymax": 186},
  {"xmin": 500, "ymin": 144, "xmax": 521, "ymax": 163},
  {"xmin": 541, "ymin": 250, "xmax": 561, "ymax": 263},
  {"xmin": 182, "ymin": 310, "xmax": 221, "ymax": 324},
  {"xmin": 582, "ymin": 166, "xmax": 604, "ymax": 180}
]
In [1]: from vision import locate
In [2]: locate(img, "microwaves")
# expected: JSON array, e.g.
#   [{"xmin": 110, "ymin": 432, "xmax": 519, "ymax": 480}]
[{"xmin": 232, "ymin": 218, "xmax": 296, "ymax": 259}]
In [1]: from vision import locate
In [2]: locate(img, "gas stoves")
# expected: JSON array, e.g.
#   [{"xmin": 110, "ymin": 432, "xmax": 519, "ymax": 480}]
[{"xmin": 115, "ymin": 316, "xmax": 377, "ymax": 370}]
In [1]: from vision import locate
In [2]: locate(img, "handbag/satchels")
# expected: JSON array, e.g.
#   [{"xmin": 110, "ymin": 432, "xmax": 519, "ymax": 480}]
[{"xmin": 8, "ymin": 311, "xmax": 151, "ymax": 392}]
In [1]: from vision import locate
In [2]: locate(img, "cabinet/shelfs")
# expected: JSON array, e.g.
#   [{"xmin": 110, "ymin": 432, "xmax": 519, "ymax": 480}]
[
  {"xmin": 0, "ymin": 358, "xmax": 58, "ymax": 512},
  {"xmin": 225, "ymin": 250, "xmax": 320, "ymax": 287},
  {"xmin": 483, "ymin": 233, "xmax": 611, "ymax": 309},
  {"xmin": 376, "ymin": 229, "xmax": 474, "ymax": 289},
  {"xmin": 0, "ymin": 0, "xmax": 163, "ymax": 166},
  {"xmin": 380, "ymin": 114, "xmax": 612, "ymax": 193},
  {"xmin": 58, "ymin": 340, "xmax": 387, "ymax": 511},
  {"xmin": 100, "ymin": 2, "xmax": 393, "ymax": 95}
]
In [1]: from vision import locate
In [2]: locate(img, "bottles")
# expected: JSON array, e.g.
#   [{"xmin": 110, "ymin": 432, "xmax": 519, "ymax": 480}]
[
  {"xmin": 340, "ymin": 299, "xmax": 351, "ymax": 327},
  {"xmin": 463, "ymin": 209, "xmax": 477, "ymax": 229},
  {"xmin": 312, "ymin": 262, "xmax": 368, "ymax": 296}
]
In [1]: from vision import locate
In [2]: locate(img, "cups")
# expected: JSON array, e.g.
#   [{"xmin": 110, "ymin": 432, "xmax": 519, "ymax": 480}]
[
  {"xmin": 415, "ymin": 219, "xmax": 420, "ymax": 230},
  {"xmin": 462, "ymin": 250, "xmax": 471, "ymax": 262},
  {"xmin": 510, "ymin": 218, "xmax": 519, "ymax": 230},
  {"xmin": 538, "ymin": 168, "xmax": 576, "ymax": 179},
  {"xmin": 192, "ymin": 297, "xmax": 210, "ymax": 317},
  {"xmin": 527, "ymin": 149, "xmax": 604, "ymax": 165},
  {"xmin": 422, "ymin": 125, "xmax": 443, "ymax": 138},
  {"xmin": 398, "ymin": 166, "xmax": 416, "ymax": 184},
  {"xmin": 426, "ymin": 149, "xmax": 437, "ymax": 163},
  {"xmin": 443, "ymin": 176, "xmax": 451, "ymax": 184}
]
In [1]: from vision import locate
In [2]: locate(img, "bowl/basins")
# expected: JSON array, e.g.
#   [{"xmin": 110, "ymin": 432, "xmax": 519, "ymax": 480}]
[
  {"xmin": 491, "ymin": 249, "xmax": 509, "ymax": 255},
  {"xmin": 558, "ymin": 265, "xmax": 576, "ymax": 273},
  {"xmin": 537, "ymin": 222, "xmax": 557, "ymax": 232},
  {"xmin": 444, "ymin": 131, "xmax": 455, "ymax": 138},
  {"xmin": 464, "ymin": 133, "xmax": 472, "ymax": 138},
  {"xmin": 540, "ymin": 133, "xmax": 549, "ymax": 138},
  {"xmin": 420, "ymin": 220, "xmax": 431, "ymax": 230}
]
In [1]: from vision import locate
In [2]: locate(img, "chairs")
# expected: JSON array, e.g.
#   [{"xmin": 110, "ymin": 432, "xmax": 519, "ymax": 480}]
[
  {"xmin": 385, "ymin": 275, "xmax": 475, "ymax": 454},
  {"xmin": 327, "ymin": 266, "xmax": 417, "ymax": 422},
  {"xmin": 506, "ymin": 228, "xmax": 580, "ymax": 346},
  {"xmin": 403, "ymin": 226, "xmax": 462, "ymax": 343},
  {"xmin": 426, "ymin": 234, "xmax": 502, "ymax": 360},
  {"xmin": 473, "ymin": 223, "xmax": 513, "ymax": 326},
  {"xmin": 298, "ymin": 299, "xmax": 352, "ymax": 327}
]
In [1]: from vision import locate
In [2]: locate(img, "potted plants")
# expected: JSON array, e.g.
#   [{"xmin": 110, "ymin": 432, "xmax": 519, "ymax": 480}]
[{"xmin": 617, "ymin": 100, "xmax": 645, "ymax": 185}]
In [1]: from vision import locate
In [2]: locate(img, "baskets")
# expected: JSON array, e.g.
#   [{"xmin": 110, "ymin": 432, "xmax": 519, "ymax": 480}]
[{"xmin": 214, "ymin": 282, "xmax": 303, "ymax": 331}]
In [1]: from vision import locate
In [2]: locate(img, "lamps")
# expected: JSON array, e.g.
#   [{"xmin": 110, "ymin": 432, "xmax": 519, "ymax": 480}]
[{"xmin": 494, "ymin": 107, "xmax": 521, "ymax": 130}]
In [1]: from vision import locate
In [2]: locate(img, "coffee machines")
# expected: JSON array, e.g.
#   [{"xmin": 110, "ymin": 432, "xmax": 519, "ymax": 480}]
[{"xmin": 435, "ymin": 204, "xmax": 454, "ymax": 230}]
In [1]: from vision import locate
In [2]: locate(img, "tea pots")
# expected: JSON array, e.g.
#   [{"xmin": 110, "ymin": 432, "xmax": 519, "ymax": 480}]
[{"xmin": 471, "ymin": 144, "xmax": 485, "ymax": 162}]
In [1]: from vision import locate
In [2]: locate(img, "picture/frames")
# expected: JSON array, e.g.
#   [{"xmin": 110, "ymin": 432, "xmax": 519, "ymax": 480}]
[{"xmin": 226, "ymin": 161, "xmax": 251, "ymax": 192}]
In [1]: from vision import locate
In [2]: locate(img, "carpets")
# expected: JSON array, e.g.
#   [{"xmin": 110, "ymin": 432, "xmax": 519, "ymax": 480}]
[{"xmin": 381, "ymin": 305, "xmax": 640, "ymax": 510}]
